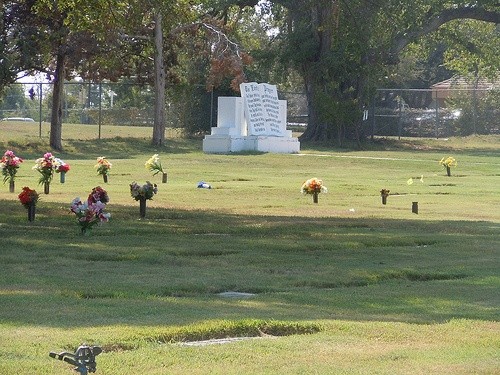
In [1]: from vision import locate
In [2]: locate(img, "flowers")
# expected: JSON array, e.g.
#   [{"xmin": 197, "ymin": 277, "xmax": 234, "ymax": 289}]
[
  {"xmin": 0, "ymin": 149, "xmax": 23, "ymax": 183},
  {"xmin": 32, "ymin": 152, "xmax": 70, "ymax": 187},
  {"xmin": 440, "ymin": 157, "xmax": 458, "ymax": 169},
  {"xmin": 146, "ymin": 153, "xmax": 165, "ymax": 175},
  {"xmin": 94, "ymin": 156, "xmax": 112, "ymax": 176},
  {"xmin": 18, "ymin": 186, "xmax": 38, "ymax": 209},
  {"xmin": 301, "ymin": 178, "xmax": 327, "ymax": 195},
  {"xmin": 130, "ymin": 181, "xmax": 158, "ymax": 200},
  {"xmin": 380, "ymin": 187, "xmax": 390, "ymax": 195},
  {"xmin": 71, "ymin": 186, "xmax": 111, "ymax": 236}
]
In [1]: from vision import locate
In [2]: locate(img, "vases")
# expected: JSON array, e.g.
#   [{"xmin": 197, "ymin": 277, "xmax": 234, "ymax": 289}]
[
  {"xmin": 61, "ymin": 171, "xmax": 65, "ymax": 182},
  {"xmin": 45, "ymin": 181, "xmax": 50, "ymax": 194},
  {"xmin": 28, "ymin": 205, "xmax": 36, "ymax": 221},
  {"xmin": 446, "ymin": 166, "xmax": 450, "ymax": 176},
  {"xmin": 104, "ymin": 175, "xmax": 107, "ymax": 183},
  {"xmin": 313, "ymin": 193, "xmax": 319, "ymax": 203},
  {"xmin": 381, "ymin": 188, "xmax": 387, "ymax": 204},
  {"xmin": 140, "ymin": 200, "xmax": 146, "ymax": 218},
  {"xmin": 162, "ymin": 172, "xmax": 167, "ymax": 182},
  {"xmin": 9, "ymin": 181, "xmax": 14, "ymax": 192}
]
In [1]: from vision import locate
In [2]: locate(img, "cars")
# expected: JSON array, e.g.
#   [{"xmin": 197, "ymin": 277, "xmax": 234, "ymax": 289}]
[
  {"xmin": 1, "ymin": 117, "xmax": 34, "ymax": 122},
  {"xmin": 440, "ymin": 108, "xmax": 466, "ymax": 128},
  {"xmin": 415, "ymin": 109, "xmax": 436, "ymax": 127}
]
